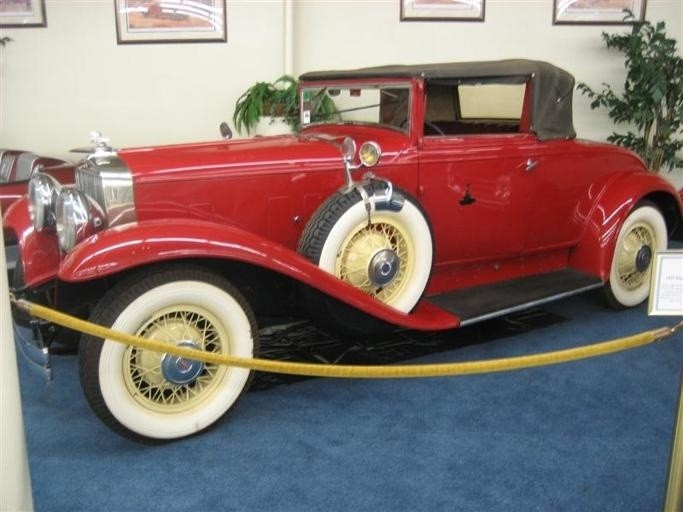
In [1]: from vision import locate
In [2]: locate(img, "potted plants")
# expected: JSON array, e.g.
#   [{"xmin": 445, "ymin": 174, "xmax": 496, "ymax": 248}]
[{"xmin": 232, "ymin": 75, "xmax": 343, "ymax": 138}]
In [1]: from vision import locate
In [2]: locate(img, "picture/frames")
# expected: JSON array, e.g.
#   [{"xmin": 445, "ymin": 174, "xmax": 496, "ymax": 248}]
[
  {"xmin": 0, "ymin": 0, "xmax": 47, "ymax": 27},
  {"xmin": 114, "ymin": 0, "xmax": 227, "ymax": 44},
  {"xmin": 647, "ymin": 250, "xmax": 683, "ymax": 316},
  {"xmin": 552, "ymin": 0, "xmax": 646, "ymax": 24},
  {"xmin": 400, "ymin": 0, "xmax": 485, "ymax": 21}
]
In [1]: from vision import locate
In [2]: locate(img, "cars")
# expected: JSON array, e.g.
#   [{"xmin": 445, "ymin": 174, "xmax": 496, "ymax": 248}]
[{"xmin": 0, "ymin": 53, "xmax": 683, "ymax": 452}]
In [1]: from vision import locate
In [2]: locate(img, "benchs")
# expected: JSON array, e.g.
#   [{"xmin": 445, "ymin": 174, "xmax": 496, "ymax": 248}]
[
  {"xmin": 0, "ymin": 148, "xmax": 75, "ymax": 246},
  {"xmin": 425, "ymin": 122, "xmax": 520, "ymax": 135}
]
